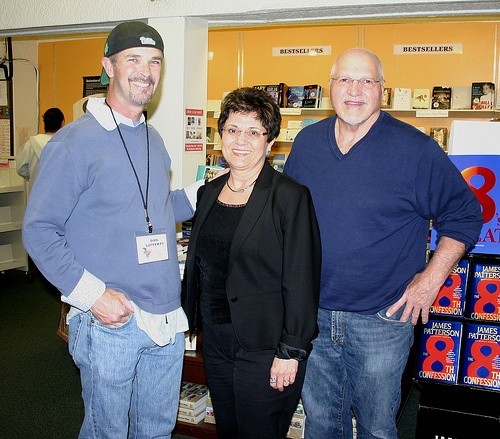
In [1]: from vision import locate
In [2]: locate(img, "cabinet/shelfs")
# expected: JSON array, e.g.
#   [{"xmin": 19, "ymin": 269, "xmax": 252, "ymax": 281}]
[{"xmin": 0, "ymin": 108, "xmax": 500, "ymax": 439}]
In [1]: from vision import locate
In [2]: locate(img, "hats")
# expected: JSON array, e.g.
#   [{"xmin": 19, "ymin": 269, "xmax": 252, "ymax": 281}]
[{"xmin": 100, "ymin": 22, "xmax": 165, "ymax": 86}]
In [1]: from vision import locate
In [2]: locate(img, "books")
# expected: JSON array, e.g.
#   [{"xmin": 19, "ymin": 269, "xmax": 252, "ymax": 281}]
[
  {"xmin": 253, "ymin": 82, "xmax": 322, "ymax": 109},
  {"xmin": 174, "ymin": 220, "xmax": 197, "ymax": 350},
  {"xmin": 286, "ymin": 399, "xmax": 306, "ymax": 439},
  {"xmin": 352, "ymin": 417, "xmax": 357, "ymax": 439},
  {"xmin": 266, "ymin": 119, "xmax": 318, "ymax": 173},
  {"xmin": 196, "ymin": 127, "xmax": 229, "ymax": 181},
  {"xmin": 418, "ymin": 260, "xmax": 500, "ymax": 391},
  {"xmin": 177, "ymin": 382, "xmax": 216, "ymax": 425},
  {"xmin": 381, "ymin": 81, "xmax": 496, "ymax": 109},
  {"xmin": 429, "ymin": 127, "xmax": 447, "ymax": 150}
]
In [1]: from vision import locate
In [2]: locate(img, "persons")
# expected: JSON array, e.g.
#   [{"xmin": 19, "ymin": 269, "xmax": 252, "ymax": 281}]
[
  {"xmin": 283, "ymin": 49, "xmax": 484, "ymax": 439},
  {"xmin": 182, "ymin": 86, "xmax": 322, "ymax": 439},
  {"xmin": 16, "ymin": 108, "xmax": 64, "ymax": 193},
  {"xmin": 480, "ymin": 83, "xmax": 491, "ymax": 101},
  {"xmin": 189, "ymin": 132, "xmax": 196, "ymax": 139},
  {"xmin": 20, "ymin": 21, "xmax": 230, "ymax": 439}
]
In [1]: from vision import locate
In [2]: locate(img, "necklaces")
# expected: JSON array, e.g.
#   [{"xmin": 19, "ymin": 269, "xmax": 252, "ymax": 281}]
[{"xmin": 226, "ymin": 179, "xmax": 256, "ymax": 192}]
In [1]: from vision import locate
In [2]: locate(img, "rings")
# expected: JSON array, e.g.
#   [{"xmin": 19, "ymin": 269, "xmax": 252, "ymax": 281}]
[
  {"xmin": 284, "ymin": 381, "xmax": 289, "ymax": 382},
  {"xmin": 270, "ymin": 377, "xmax": 275, "ymax": 383}
]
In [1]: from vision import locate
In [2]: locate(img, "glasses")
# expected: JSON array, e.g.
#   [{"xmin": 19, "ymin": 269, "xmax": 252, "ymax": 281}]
[
  {"xmin": 330, "ymin": 77, "xmax": 381, "ymax": 89},
  {"xmin": 222, "ymin": 126, "xmax": 268, "ymax": 139}
]
